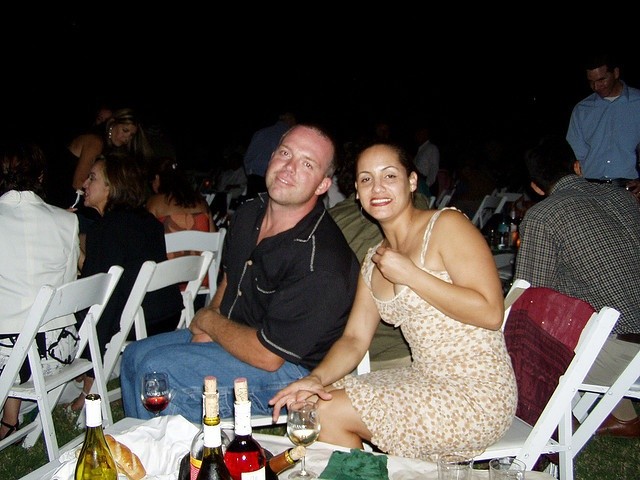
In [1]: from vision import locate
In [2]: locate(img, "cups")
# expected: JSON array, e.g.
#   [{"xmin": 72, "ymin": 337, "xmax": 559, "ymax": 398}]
[
  {"xmin": 488, "ymin": 458, "xmax": 527, "ymax": 480},
  {"xmin": 437, "ymin": 451, "xmax": 473, "ymax": 479}
]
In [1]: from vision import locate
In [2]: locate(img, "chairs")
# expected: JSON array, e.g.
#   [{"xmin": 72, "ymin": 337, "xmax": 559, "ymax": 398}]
[
  {"xmin": 449, "ymin": 278, "xmax": 621, "ymax": 480},
  {"xmin": 72, "ymin": 250, "xmax": 219, "ymax": 431},
  {"xmin": 164, "ymin": 226, "xmax": 227, "ymax": 330},
  {"xmin": 572, "ymin": 349, "xmax": 639, "ymax": 462},
  {"xmin": 199, "ymin": 351, "xmax": 371, "ymax": 430},
  {"xmin": 0, "ymin": 264, "xmax": 124, "ymax": 463}
]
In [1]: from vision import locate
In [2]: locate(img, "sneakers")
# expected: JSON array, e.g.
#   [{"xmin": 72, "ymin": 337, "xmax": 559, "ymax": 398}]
[{"xmin": 55, "ymin": 403, "xmax": 79, "ymax": 424}]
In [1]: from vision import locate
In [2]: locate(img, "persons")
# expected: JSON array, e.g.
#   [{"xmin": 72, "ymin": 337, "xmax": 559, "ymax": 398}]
[
  {"xmin": 95, "ymin": 107, "xmax": 115, "ymax": 125},
  {"xmin": 375, "ymin": 121, "xmax": 394, "ymax": 142},
  {"xmin": 147, "ymin": 156, "xmax": 215, "ymax": 313},
  {"xmin": 217, "ymin": 152, "xmax": 247, "ymax": 214},
  {"xmin": 269, "ymin": 143, "xmax": 518, "ymax": 452},
  {"xmin": 244, "ymin": 111, "xmax": 296, "ymax": 198},
  {"xmin": 413, "ymin": 126, "xmax": 440, "ymax": 207},
  {"xmin": 564, "ymin": 57, "xmax": 639, "ymax": 200},
  {"xmin": 1, "ymin": 143, "xmax": 79, "ymax": 446},
  {"xmin": 119, "ymin": 118, "xmax": 368, "ymax": 426},
  {"xmin": 54, "ymin": 108, "xmax": 150, "ymax": 209},
  {"xmin": 511, "ymin": 136, "xmax": 640, "ymax": 437},
  {"xmin": 62, "ymin": 152, "xmax": 184, "ymax": 415}
]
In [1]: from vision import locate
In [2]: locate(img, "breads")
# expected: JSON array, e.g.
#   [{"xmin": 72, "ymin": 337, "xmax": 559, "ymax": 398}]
[{"xmin": 88, "ymin": 434, "xmax": 145, "ymax": 476}]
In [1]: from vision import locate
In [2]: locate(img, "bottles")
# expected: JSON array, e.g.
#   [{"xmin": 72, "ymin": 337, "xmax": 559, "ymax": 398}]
[
  {"xmin": 227, "ymin": 379, "xmax": 262, "ymax": 448},
  {"xmin": 489, "ymin": 230, "xmax": 495, "ymax": 250},
  {"xmin": 73, "ymin": 393, "xmax": 119, "ymax": 480},
  {"xmin": 195, "ymin": 397, "xmax": 233, "ymax": 480},
  {"xmin": 223, "ymin": 400, "xmax": 266, "ymax": 480},
  {"xmin": 190, "ymin": 396, "xmax": 216, "ymax": 480},
  {"xmin": 190, "ymin": 375, "xmax": 231, "ymax": 446},
  {"xmin": 265, "ymin": 446, "xmax": 305, "ymax": 480},
  {"xmin": 503, "ymin": 232, "xmax": 509, "ymax": 247},
  {"xmin": 509, "ymin": 206, "xmax": 517, "ymax": 232}
]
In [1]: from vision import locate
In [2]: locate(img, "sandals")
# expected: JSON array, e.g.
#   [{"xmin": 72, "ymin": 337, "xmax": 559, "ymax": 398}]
[{"xmin": 0, "ymin": 420, "xmax": 19, "ymax": 441}]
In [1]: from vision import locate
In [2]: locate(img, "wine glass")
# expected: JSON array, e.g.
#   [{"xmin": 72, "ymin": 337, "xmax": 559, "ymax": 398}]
[
  {"xmin": 140, "ymin": 372, "xmax": 170, "ymax": 417},
  {"xmin": 286, "ymin": 401, "xmax": 320, "ymax": 479}
]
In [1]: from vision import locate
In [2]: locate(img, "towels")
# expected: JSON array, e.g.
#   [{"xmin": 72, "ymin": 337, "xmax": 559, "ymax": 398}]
[{"xmin": 48, "ymin": 413, "xmax": 201, "ymax": 479}]
